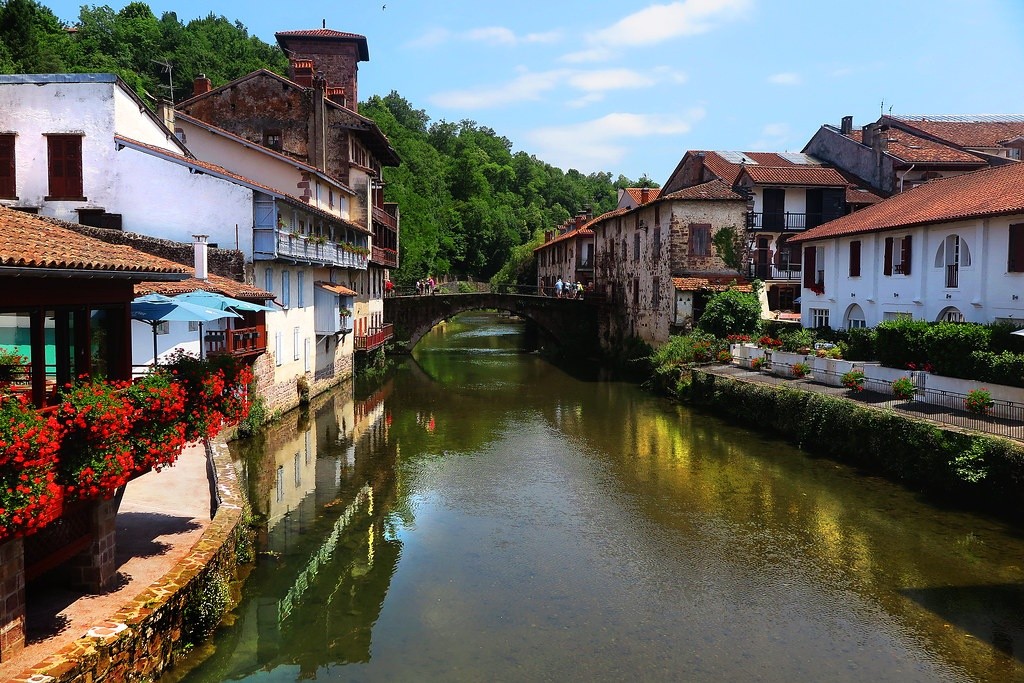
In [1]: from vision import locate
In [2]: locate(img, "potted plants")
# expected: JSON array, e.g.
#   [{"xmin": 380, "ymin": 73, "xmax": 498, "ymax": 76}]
[{"xmin": 278, "ymin": 219, "xmax": 287, "ymax": 228}]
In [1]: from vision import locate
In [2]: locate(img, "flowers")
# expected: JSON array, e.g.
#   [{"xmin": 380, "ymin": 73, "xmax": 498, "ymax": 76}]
[
  {"xmin": 841, "ymin": 369, "xmax": 865, "ymax": 393},
  {"xmin": 717, "ymin": 351, "xmax": 733, "ymax": 361},
  {"xmin": 751, "ymin": 357, "xmax": 765, "ymax": 370},
  {"xmin": 0, "ymin": 345, "xmax": 254, "ymax": 541},
  {"xmin": 891, "ymin": 376, "xmax": 916, "ymax": 402},
  {"xmin": 384, "ymin": 248, "xmax": 397, "ymax": 257},
  {"xmin": 790, "ymin": 360, "xmax": 811, "ymax": 378},
  {"xmin": 963, "ymin": 388, "xmax": 994, "ymax": 414}
]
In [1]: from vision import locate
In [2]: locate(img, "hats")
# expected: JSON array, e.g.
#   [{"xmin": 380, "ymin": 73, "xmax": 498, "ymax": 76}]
[
  {"xmin": 577, "ymin": 281, "xmax": 581, "ymax": 283},
  {"xmin": 558, "ymin": 279, "xmax": 562, "ymax": 282}
]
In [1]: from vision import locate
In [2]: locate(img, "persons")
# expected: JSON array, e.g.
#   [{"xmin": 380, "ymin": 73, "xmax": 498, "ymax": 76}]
[
  {"xmin": 539, "ymin": 279, "xmax": 547, "ymax": 296},
  {"xmin": 414, "ymin": 277, "xmax": 434, "ymax": 296},
  {"xmin": 555, "ymin": 278, "xmax": 584, "ymax": 300},
  {"xmin": 386, "ymin": 280, "xmax": 396, "ymax": 296}
]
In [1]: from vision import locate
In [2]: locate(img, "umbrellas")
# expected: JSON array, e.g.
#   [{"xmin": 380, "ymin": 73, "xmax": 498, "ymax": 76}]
[
  {"xmin": 171, "ymin": 286, "xmax": 276, "ymax": 365},
  {"xmin": 793, "ymin": 297, "xmax": 801, "ymax": 305},
  {"xmin": 49, "ymin": 292, "xmax": 244, "ymax": 375}
]
